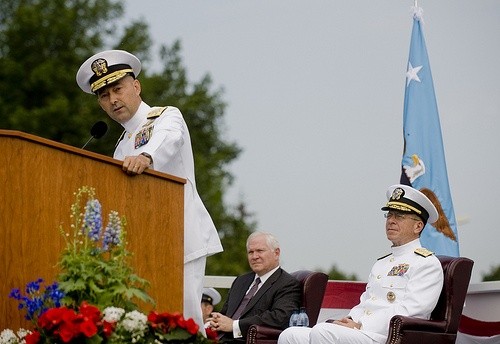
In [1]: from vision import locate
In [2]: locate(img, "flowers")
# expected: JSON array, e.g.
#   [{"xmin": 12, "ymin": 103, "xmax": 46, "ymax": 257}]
[{"xmin": 0, "ymin": 185, "xmax": 212, "ymax": 344}]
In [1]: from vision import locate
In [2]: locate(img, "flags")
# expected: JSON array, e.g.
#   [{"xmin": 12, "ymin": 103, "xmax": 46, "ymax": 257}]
[{"xmin": 400, "ymin": 8, "xmax": 465, "ymax": 312}]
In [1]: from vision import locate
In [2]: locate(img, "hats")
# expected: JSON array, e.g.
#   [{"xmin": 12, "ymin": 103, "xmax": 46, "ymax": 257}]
[
  {"xmin": 76, "ymin": 50, "xmax": 141, "ymax": 95},
  {"xmin": 381, "ymin": 185, "xmax": 439, "ymax": 224},
  {"xmin": 201, "ymin": 287, "xmax": 221, "ymax": 306}
]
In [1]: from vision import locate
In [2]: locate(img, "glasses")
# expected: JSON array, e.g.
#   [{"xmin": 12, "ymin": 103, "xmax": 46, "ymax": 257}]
[{"xmin": 384, "ymin": 211, "xmax": 420, "ymax": 221}]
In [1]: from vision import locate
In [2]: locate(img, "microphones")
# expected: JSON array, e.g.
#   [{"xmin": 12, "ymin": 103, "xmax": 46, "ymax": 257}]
[{"xmin": 82, "ymin": 121, "xmax": 108, "ymax": 149}]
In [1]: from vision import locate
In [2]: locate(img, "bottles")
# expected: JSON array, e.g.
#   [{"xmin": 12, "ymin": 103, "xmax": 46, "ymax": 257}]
[
  {"xmin": 297, "ymin": 307, "xmax": 309, "ymax": 327},
  {"xmin": 289, "ymin": 307, "xmax": 299, "ymax": 327}
]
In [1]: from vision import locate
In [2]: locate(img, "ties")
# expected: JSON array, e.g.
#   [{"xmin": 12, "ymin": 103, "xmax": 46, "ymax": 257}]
[{"xmin": 230, "ymin": 278, "xmax": 261, "ymax": 320}]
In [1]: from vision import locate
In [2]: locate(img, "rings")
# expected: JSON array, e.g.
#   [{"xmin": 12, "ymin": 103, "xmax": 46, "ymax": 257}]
[{"xmin": 217, "ymin": 323, "xmax": 218, "ymax": 325}]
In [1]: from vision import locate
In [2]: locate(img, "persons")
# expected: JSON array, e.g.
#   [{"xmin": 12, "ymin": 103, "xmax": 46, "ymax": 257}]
[
  {"xmin": 206, "ymin": 232, "xmax": 302, "ymax": 344},
  {"xmin": 76, "ymin": 50, "xmax": 224, "ymax": 337},
  {"xmin": 277, "ymin": 184, "xmax": 444, "ymax": 344},
  {"xmin": 201, "ymin": 287, "xmax": 221, "ymax": 338}
]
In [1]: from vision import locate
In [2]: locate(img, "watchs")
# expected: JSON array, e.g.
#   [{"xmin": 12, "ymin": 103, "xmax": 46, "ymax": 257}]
[{"xmin": 141, "ymin": 152, "xmax": 152, "ymax": 164}]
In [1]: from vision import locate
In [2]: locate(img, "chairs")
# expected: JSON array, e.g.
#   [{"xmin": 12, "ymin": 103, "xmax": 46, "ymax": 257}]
[
  {"xmin": 385, "ymin": 254, "xmax": 475, "ymax": 344},
  {"xmin": 246, "ymin": 270, "xmax": 329, "ymax": 344}
]
